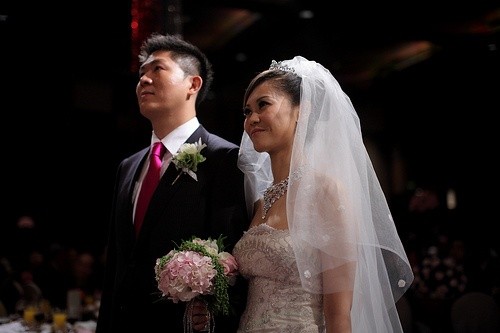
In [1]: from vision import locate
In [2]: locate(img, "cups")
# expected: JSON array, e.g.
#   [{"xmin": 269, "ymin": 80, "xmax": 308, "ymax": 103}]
[
  {"xmin": 24, "ymin": 305, "xmax": 38, "ymax": 324},
  {"xmin": 54, "ymin": 310, "xmax": 67, "ymax": 328}
]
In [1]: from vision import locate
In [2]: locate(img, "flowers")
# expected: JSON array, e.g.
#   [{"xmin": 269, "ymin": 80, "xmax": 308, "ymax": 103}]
[
  {"xmin": 172, "ymin": 140, "xmax": 206, "ymax": 179},
  {"xmin": 153, "ymin": 237, "xmax": 237, "ymax": 301}
]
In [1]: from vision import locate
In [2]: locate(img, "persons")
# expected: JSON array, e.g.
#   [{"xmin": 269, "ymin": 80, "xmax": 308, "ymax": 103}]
[
  {"xmin": 95, "ymin": 33, "xmax": 254, "ymax": 333},
  {"xmin": 233, "ymin": 55, "xmax": 415, "ymax": 332},
  {"xmin": 1, "ymin": 250, "xmax": 106, "ymax": 316}
]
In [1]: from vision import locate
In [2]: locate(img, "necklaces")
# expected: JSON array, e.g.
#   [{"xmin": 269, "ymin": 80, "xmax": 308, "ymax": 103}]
[{"xmin": 261, "ymin": 166, "xmax": 302, "ymax": 219}]
